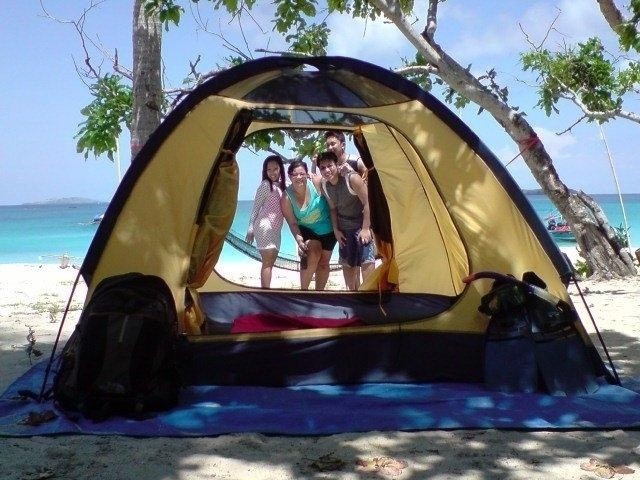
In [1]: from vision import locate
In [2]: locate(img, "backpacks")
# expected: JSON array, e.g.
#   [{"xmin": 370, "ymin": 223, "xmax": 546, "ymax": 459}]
[{"xmin": 45, "ymin": 272, "xmax": 192, "ymax": 423}]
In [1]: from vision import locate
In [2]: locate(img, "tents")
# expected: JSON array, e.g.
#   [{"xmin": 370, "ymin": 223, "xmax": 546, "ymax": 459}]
[{"xmin": 37, "ymin": 55, "xmax": 622, "ymax": 388}]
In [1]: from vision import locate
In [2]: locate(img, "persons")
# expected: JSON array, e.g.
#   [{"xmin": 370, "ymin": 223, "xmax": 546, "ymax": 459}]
[
  {"xmin": 245, "ymin": 156, "xmax": 287, "ymax": 288},
  {"xmin": 316, "ymin": 151, "xmax": 372, "ymax": 291},
  {"xmin": 280, "ymin": 161, "xmax": 337, "ymax": 291},
  {"xmin": 321, "ymin": 131, "xmax": 377, "ymax": 291}
]
